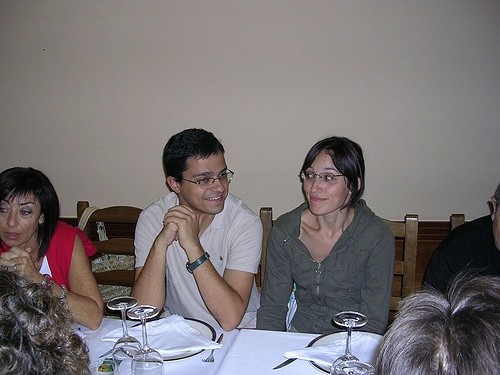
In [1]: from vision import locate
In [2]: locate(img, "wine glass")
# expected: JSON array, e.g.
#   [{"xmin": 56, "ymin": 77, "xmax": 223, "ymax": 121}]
[
  {"xmin": 127, "ymin": 304, "xmax": 163, "ymax": 375},
  {"xmin": 330, "ymin": 311, "xmax": 368, "ymax": 375},
  {"xmin": 107, "ymin": 295, "xmax": 143, "ymax": 375},
  {"xmin": 334, "ymin": 360, "xmax": 375, "ymax": 375}
]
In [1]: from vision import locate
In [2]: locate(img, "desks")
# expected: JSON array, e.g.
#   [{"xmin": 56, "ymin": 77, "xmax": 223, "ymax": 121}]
[
  {"xmin": 72, "ymin": 319, "xmax": 238, "ymax": 375},
  {"xmin": 216, "ymin": 328, "xmax": 325, "ymax": 375}
]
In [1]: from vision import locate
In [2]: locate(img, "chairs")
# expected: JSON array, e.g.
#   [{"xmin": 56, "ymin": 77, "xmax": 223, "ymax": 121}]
[
  {"xmin": 76, "ymin": 200, "xmax": 143, "ymax": 317},
  {"xmin": 259, "ymin": 208, "xmax": 418, "ymax": 311}
]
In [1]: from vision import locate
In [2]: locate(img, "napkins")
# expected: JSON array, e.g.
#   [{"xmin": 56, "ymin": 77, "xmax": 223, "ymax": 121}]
[
  {"xmin": 283, "ymin": 333, "xmax": 381, "ymax": 367},
  {"xmin": 99, "ymin": 314, "xmax": 224, "ymax": 355}
]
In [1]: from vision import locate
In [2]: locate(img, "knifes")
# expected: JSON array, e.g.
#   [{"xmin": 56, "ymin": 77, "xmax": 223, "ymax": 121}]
[
  {"xmin": 273, "ymin": 358, "xmax": 297, "ymax": 370},
  {"xmin": 99, "ymin": 349, "xmax": 113, "ymax": 358}
]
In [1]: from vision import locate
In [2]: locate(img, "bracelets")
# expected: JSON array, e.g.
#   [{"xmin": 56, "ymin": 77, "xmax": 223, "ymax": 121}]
[
  {"xmin": 40, "ymin": 274, "xmax": 52, "ymax": 289},
  {"xmin": 57, "ymin": 284, "xmax": 67, "ymax": 302}
]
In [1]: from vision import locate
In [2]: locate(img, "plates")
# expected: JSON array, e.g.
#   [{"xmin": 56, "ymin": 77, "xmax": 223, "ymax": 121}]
[
  {"xmin": 306, "ymin": 329, "xmax": 385, "ymax": 375},
  {"xmin": 119, "ymin": 316, "xmax": 217, "ymax": 363}
]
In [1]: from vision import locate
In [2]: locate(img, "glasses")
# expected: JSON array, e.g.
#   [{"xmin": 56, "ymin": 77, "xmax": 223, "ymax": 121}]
[
  {"xmin": 182, "ymin": 169, "xmax": 234, "ymax": 187},
  {"xmin": 299, "ymin": 171, "xmax": 346, "ymax": 183}
]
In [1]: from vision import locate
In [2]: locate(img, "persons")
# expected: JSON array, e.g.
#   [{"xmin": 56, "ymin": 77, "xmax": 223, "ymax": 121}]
[
  {"xmin": 128, "ymin": 128, "xmax": 263, "ymax": 332},
  {"xmin": 0, "ymin": 167, "xmax": 105, "ymax": 332},
  {"xmin": 374, "ymin": 270, "xmax": 500, "ymax": 375},
  {"xmin": 418, "ymin": 180, "xmax": 499, "ymax": 296},
  {"xmin": 255, "ymin": 137, "xmax": 394, "ymax": 335}
]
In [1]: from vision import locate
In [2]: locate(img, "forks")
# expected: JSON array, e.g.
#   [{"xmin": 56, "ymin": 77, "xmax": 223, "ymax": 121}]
[{"xmin": 202, "ymin": 332, "xmax": 224, "ymax": 363}]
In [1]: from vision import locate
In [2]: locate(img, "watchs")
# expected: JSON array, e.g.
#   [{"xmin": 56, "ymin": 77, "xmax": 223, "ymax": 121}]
[{"xmin": 185, "ymin": 251, "xmax": 210, "ymax": 275}]
